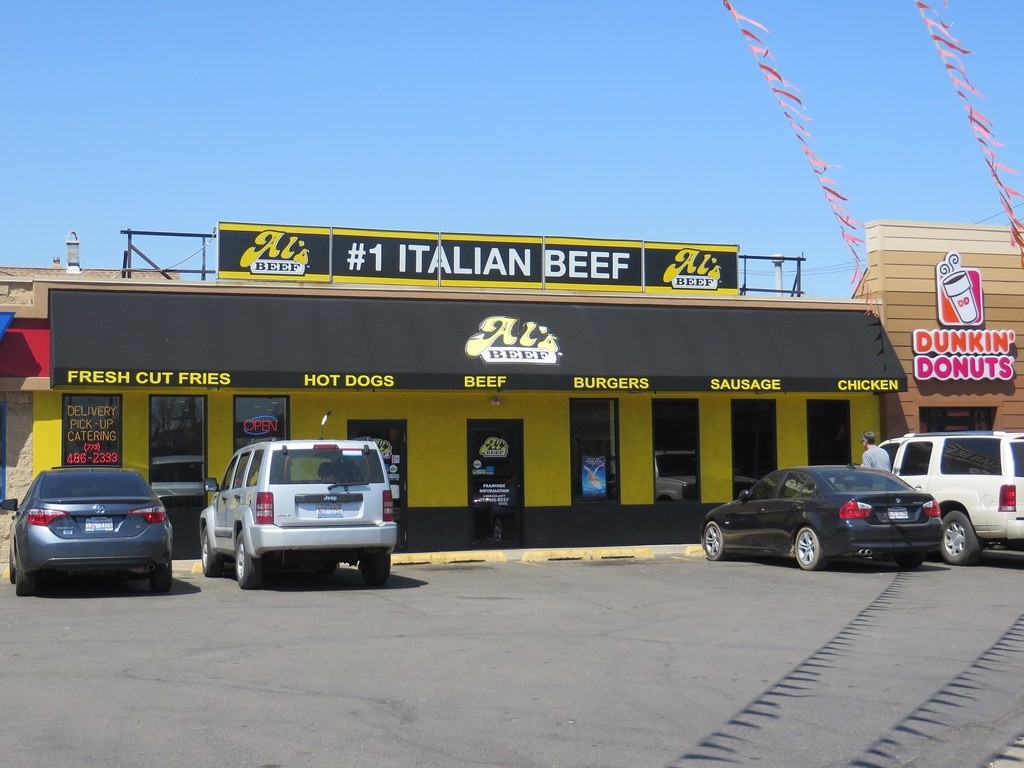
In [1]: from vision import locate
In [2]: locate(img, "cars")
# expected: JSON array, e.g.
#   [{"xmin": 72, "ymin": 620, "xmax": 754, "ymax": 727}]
[
  {"xmin": 700, "ymin": 462, "xmax": 943, "ymax": 575},
  {"xmin": 1, "ymin": 465, "xmax": 174, "ymax": 597}
]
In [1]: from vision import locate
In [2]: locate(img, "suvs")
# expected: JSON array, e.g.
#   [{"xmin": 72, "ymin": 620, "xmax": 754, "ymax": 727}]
[
  {"xmin": 873, "ymin": 430, "xmax": 1023, "ymax": 568},
  {"xmin": 196, "ymin": 436, "xmax": 398, "ymax": 590},
  {"xmin": 652, "ymin": 449, "xmax": 758, "ymax": 506}
]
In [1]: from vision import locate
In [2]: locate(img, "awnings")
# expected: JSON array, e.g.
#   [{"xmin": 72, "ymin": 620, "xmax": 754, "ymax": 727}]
[{"xmin": 42, "ymin": 287, "xmax": 911, "ymax": 397}]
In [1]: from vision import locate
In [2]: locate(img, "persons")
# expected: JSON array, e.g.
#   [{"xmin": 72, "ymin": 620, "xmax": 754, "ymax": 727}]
[{"xmin": 858, "ymin": 431, "xmax": 891, "ymax": 474}]
